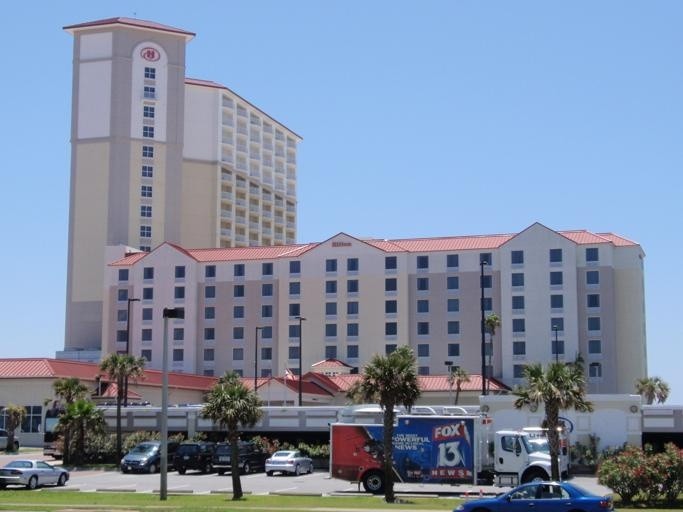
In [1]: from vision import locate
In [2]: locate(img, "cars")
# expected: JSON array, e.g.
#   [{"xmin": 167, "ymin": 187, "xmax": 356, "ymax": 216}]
[
  {"xmin": 452, "ymin": 481, "xmax": 614, "ymax": 512},
  {"xmin": 0, "ymin": 428, "xmax": 20, "ymax": 451},
  {"xmin": 0, "ymin": 459, "xmax": 70, "ymax": 489},
  {"xmin": 120, "ymin": 441, "xmax": 315, "ymax": 476}
]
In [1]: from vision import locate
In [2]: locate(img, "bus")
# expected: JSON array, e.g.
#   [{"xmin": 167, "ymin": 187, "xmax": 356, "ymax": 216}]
[{"xmin": 42, "ymin": 402, "xmax": 150, "ymax": 460}]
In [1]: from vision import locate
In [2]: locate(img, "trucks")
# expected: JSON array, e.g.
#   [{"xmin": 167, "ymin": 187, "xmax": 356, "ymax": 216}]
[{"xmin": 329, "ymin": 413, "xmax": 570, "ymax": 492}]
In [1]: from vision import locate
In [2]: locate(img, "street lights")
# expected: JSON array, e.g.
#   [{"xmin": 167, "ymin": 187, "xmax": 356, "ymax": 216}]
[
  {"xmin": 479, "ymin": 255, "xmax": 491, "ymax": 396},
  {"xmin": 124, "ymin": 298, "xmax": 140, "ymax": 408},
  {"xmin": 255, "ymin": 326, "xmax": 268, "ymax": 394},
  {"xmin": 294, "ymin": 316, "xmax": 307, "ymax": 407},
  {"xmin": 552, "ymin": 325, "xmax": 558, "ymax": 363},
  {"xmin": 160, "ymin": 308, "xmax": 178, "ymax": 499}
]
